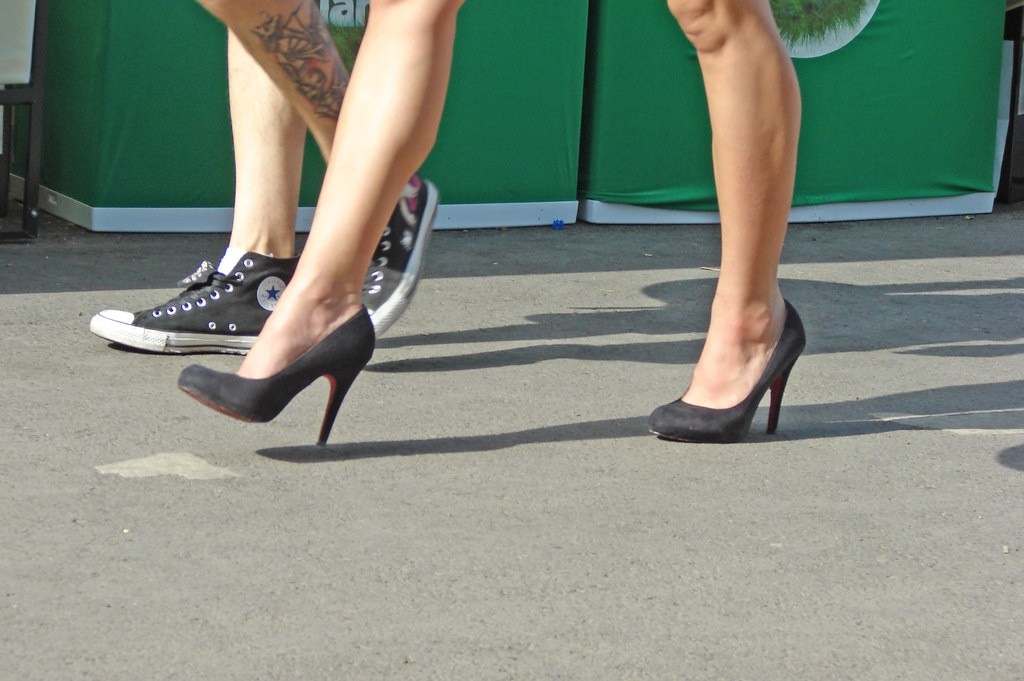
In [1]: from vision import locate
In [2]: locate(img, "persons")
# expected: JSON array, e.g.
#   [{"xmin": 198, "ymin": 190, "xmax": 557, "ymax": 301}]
[
  {"xmin": 90, "ymin": 0, "xmax": 438, "ymax": 357},
  {"xmin": 179, "ymin": 0, "xmax": 805, "ymax": 445}
]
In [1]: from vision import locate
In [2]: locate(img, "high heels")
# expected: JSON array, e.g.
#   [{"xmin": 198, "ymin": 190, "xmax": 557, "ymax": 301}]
[
  {"xmin": 179, "ymin": 304, "xmax": 376, "ymax": 446},
  {"xmin": 649, "ymin": 298, "xmax": 806, "ymax": 443}
]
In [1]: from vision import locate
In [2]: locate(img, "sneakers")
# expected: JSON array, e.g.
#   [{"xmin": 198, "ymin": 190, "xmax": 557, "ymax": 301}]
[
  {"xmin": 90, "ymin": 251, "xmax": 300, "ymax": 354},
  {"xmin": 362, "ymin": 179, "xmax": 438, "ymax": 337}
]
